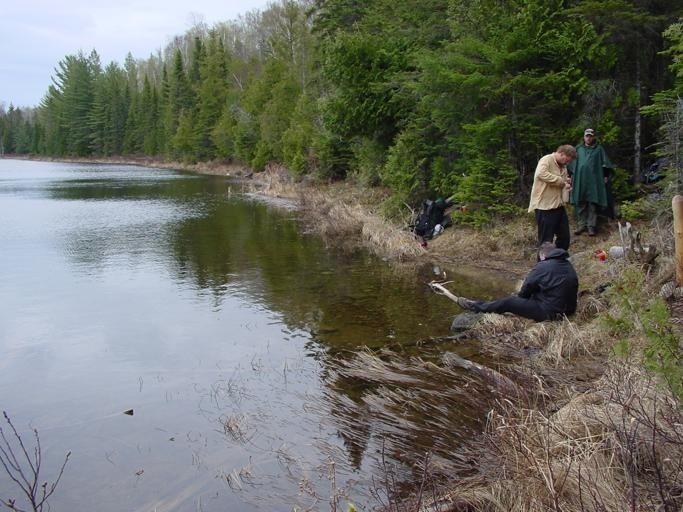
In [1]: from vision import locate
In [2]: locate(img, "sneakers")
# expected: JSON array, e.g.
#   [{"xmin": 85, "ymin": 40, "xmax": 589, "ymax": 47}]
[
  {"xmin": 574, "ymin": 229, "xmax": 595, "ymax": 236},
  {"xmin": 457, "ymin": 296, "xmax": 475, "ymax": 311}
]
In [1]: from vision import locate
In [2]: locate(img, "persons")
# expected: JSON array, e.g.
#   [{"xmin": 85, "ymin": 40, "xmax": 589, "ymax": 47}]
[
  {"xmin": 527, "ymin": 143, "xmax": 576, "ymax": 251},
  {"xmin": 566, "ymin": 128, "xmax": 616, "ymax": 237},
  {"xmin": 456, "ymin": 241, "xmax": 579, "ymax": 323}
]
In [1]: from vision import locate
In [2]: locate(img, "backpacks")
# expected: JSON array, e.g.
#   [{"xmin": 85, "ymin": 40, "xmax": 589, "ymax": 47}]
[{"xmin": 410, "ymin": 199, "xmax": 452, "ymax": 238}]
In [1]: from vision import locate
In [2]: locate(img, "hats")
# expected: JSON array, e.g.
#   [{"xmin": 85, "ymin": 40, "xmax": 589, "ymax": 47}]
[{"xmin": 584, "ymin": 129, "xmax": 594, "ymax": 136}]
